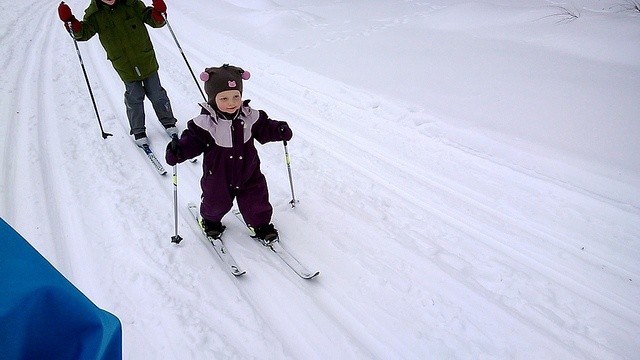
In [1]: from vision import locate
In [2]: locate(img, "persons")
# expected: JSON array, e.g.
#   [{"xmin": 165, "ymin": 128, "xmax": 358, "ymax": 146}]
[
  {"xmin": 165, "ymin": 64, "xmax": 293, "ymax": 242},
  {"xmin": 58, "ymin": 0, "xmax": 177, "ymax": 146}
]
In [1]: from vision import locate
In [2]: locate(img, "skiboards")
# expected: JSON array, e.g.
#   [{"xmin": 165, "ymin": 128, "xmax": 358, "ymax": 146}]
[
  {"xmin": 130, "ymin": 123, "xmax": 197, "ymax": 174},
  {"xmin": 187, "ymin": 201, "xmax": 319, "ymax": 282}
]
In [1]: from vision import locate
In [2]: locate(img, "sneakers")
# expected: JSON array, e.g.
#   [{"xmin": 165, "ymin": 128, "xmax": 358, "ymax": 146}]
[
  {"xmin": 254, "ymin": 224, "xmax": 278, "ymax": 242},
  {"xmin": 202, "ymin": 218, "xmax": 222, "ymax": 240},
  {"xmin": 165, "ymin": 124, "xmax": 178, "ymax": 138},
  {"xmin": 133, "ymin": 134, "xmax": 148, "ymax": 146}
]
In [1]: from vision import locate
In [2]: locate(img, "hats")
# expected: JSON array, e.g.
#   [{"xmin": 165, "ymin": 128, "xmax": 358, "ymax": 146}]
[{"xmin": 200, "ymin": 64, "xmax": 250, "ymax": 114}]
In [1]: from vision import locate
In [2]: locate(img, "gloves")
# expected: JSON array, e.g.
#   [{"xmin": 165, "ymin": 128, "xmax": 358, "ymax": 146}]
[
  {"xmin": 276, "ymin": 123, "xmax": 293, "ymax": 141},
  {"xmin": 151, "ymin": 0, "xmax": 167, "ymax": 22},
  {"xmin": 58, "ymin": 3, "xmax": 81, "ymax": 33},
  {"xmin": 165, "ymin": 139, "xmax": 188, "ymax": 166}
]
